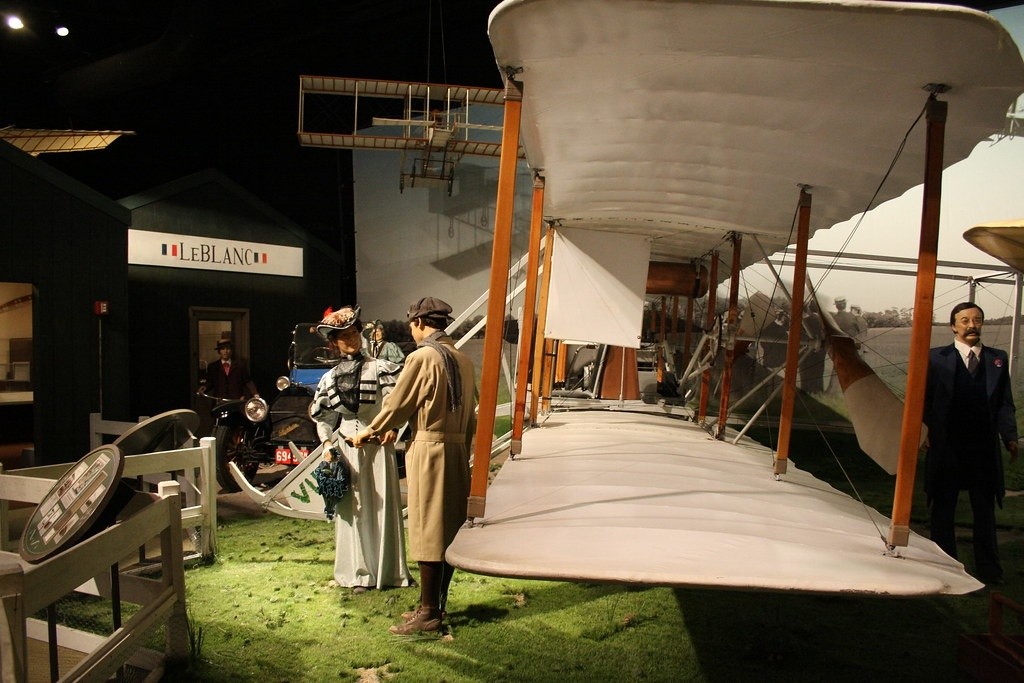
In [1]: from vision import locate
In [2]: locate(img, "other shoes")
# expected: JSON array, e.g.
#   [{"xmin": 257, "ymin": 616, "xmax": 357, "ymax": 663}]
[
  {"xmin": 991, "ymin": 576, "xmax": 1005, "ymax": 586},
  {"xmin": 353, "ymin": 586, "xmax": 366, "ymax": 593},
  {"xmin": 408, "ymin": 578, "xmax": 419, "ymax": 588}
]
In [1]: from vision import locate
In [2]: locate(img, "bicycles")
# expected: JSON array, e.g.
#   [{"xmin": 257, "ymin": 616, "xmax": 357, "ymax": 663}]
[{"xmin": 199, "ymin": 390, "xmax": 257, "ymax": 441}]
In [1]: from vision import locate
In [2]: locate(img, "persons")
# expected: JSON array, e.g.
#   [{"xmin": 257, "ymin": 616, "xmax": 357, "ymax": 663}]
[
  {"xmin": 362, "ymin": 319, "xmax": 405, "ymax": 363},
  {"xmin": 919, "ymin": 303, "xmax": 1020, "ymax": 586},
  {"xmin": 759, "ymin": 298, "xmax": 870, "ymax": 392},
  {"xmin": 351, "ymin": 296, "xmax": 474, "ymax": 634},
  {"xmin": 206, "ymin": 339, "xmax": 260, "ymax": 426},
  {"xmin": 308, "ymin": 308, "xmax": 415, "ymax": 591}
]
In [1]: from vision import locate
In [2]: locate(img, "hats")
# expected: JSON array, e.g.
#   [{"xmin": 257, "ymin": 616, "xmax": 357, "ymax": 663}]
[
  {"xmin": 214, "ymin": 339, "xmax": 235, "ymax": 350},
  {"xmin": 316, "ymin": 304, "xmax": 361, "ymax": 339},
  {"xmin": 833, "ymin": 296, "xmax": 847, "ymax": 304},
  {"xmin": 851, "ymin": 304, "xmax": 861, "ymax": 309},
  {"xmin": 407, "ymin": 296, "xmax": 455, "ymax": 323},
  {"xmin": 362, "ymin": 318, "xmax": 384, "ymax": 340}
]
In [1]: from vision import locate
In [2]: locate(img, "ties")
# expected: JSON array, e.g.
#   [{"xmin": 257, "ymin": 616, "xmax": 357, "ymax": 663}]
[
  {"xmin": 968, "ymin": 350, "xmax": 978, "ymax": 379},
  {"xmin": 223, "ymin": 362, "xmax": 231, "ymax": 375}
]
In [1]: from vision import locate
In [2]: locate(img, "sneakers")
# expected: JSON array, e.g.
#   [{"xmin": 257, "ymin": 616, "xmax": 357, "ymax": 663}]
[
  {"xmin": 390, "ymin": 604, "xmax": 443, "ymax": 635},
  {"xmin": 400, "ymin": 602, "xmax": 423, "ymax": 621}
]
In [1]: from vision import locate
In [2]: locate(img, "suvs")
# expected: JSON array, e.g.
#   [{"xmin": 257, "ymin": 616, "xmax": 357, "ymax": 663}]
[{"xmin": 210, "ymin": 319, "xmax": 417, "ymax": 494}]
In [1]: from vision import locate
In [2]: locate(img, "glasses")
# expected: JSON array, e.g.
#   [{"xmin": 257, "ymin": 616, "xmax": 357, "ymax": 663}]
[{"xmin": 955, "ymin": 317, "xmax": 983, "ymax": 325}]
